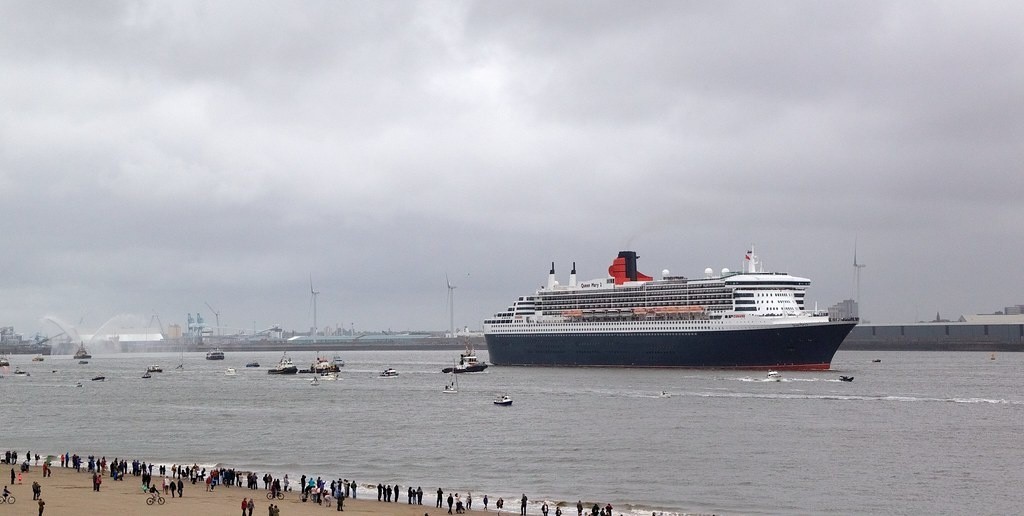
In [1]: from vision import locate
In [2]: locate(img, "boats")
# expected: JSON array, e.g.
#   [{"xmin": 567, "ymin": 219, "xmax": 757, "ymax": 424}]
[
  {"xmin": 443, "ymin": 387, "xmax": 457, "ymax": 393},
  {"xmin": 379, "ymin": 369, "xmax": 399, "ymax": 377},
  {"xmin": 443, "ymin": 342, "xmax": 488, "ymax": 373},
  {"xmin": 91, "ymin": 376, "xmax": 106, "ymax": 381},
  {"xmin": 767, "ymin": 371, "xmax": 782, "ymax": 380},
  {"xmin": 225, "ymin": 369, "xmax": 237, "ymax": 375},
  {"xmin": 662, "ymin": 390, "xmax": 671, "ymax": 398},
  {"xmin": 0, "ymin": 357, "xmax": 9, "ymax": 366},
  {"xmin": 267, "ymin": 353, "xmax": 298, "ymax": 374},
  {"xmin": 141, "ymin": 372, "xmax": 151, "ymax": 378},
  {"xmin": 872, "ymin": 359, "xmax": 881, "ymax": 363},
  {"xmin": 330, "ymin": 354, "xmax": 344, "ymax": 367},
  {"xmin": 494, "ymin": 395, "xmax": 513, "ymax": 406},
  {"xmin": 147, "ymin": 365, "xmax": 162, "ymax": 372},
  {"xmin": 839, "ymin": 375, "xmax": 854, "ymax": 382},
  {"xmin": 299, "ymin": 357, "xmax": 341, "ymax": 373},
  {"xmin": 246, "ymin": 363, "xmax": 260, "ymax": 367},
  {"xmin": 32, "ymin": 356, "xmax": 44, "ymax": 361},
  {"xmin": 74, "ymin": 341, "xmax": 92, "ymax": 359},
  {"xmin": 206, "ymin": 348, "xmax": 225, "ymax": 360},
  {"xmin": 14, "ymin": 370, "xmax": 30, "ymax": 377}
]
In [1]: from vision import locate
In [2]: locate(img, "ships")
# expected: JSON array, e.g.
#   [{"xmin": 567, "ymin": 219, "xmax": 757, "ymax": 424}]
[{"xmin": 483, "ymin": 244, "xmax": 860, "ymax": 371}]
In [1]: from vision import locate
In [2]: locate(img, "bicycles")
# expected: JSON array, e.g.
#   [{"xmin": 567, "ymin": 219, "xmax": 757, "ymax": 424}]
[
  {"xmin": 266, "ymin": 491, "xmax": 284, "ymax": 501},
  {"xmin": 146, "ymin": 494, "xmax": 165, "ymax": 505},
  {"xmin": 0, "ymin": 493, "xmax": 15, "ymax": 505},
  {"xmin": 299, "ymin": 492, "xmax": 314, "ymax": 501}
]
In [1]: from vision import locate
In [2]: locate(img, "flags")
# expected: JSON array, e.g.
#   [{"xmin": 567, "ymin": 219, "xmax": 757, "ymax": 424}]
[{"xmin": 745, "ymin": 251, "xmax": 752, "ymax": 260}]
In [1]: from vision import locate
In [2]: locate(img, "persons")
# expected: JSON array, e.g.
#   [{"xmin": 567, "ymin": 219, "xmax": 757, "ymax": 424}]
[{"xmin": 1, "ymin": 449, "xmax": 614, "ymax": 516}]
